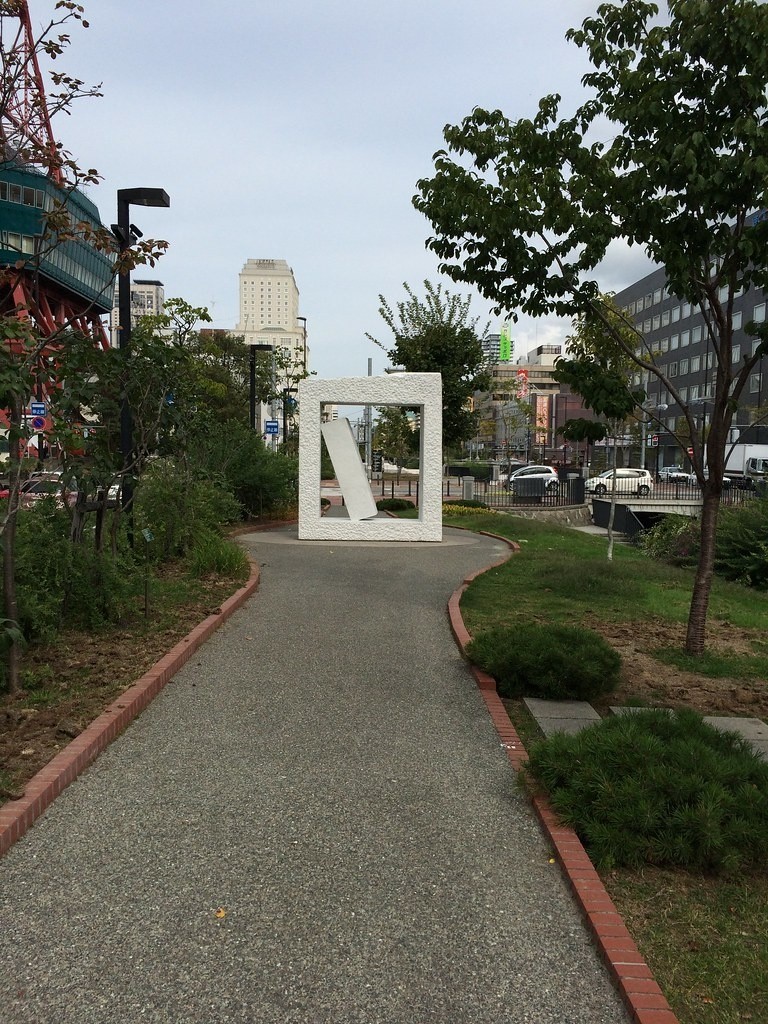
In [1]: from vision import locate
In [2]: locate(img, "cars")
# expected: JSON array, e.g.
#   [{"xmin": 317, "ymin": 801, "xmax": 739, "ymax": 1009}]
[
  {"xmin": 689, "ymin": 468, "xmax": 731, "ymax": 488},
  {"xmin": 584, "ymin": 467, "xmax": 654, "ymax": 495},
  {"xmin": 502, "ymin": 465, "xmax": 560, "ymax": 492},
  {"xmin": 658, "ymin": 466, "xmax": 690, "ymax": 483},
  {"xmin": 0, "ymin": 470, "xmax": 123, "ymax": 511}
]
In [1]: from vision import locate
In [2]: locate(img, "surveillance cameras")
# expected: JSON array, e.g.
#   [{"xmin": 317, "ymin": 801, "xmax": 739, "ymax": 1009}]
[{"xmin": 129, "ymin": 224, "xmax": 142, "ymax": 237}]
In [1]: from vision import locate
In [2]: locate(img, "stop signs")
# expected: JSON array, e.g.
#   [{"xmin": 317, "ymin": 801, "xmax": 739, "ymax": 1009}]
[
  {"xmin": 688, "ymin": 448, "xmax": 694, "ymax": 457},
  {"xmin": 502, "ymin": 440, "xmax": 506, "ymax": 445},
  {"xmin": 563, "ymin": 440, "xmax": 569, "ymax": 448}
]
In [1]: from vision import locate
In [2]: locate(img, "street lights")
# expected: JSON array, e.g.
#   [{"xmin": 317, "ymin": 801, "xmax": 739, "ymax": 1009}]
[
  {"xmin": 249, "ymin": 344, "xmax": 277, "ymax": 431},
  {"xmin": 559, "ymin": 395, "xmax": 568, "ymax": 467},
  {"xmin": 540, "ymin": 436, "xmax": 546, "ymax": 465},
  {"xmin": 116, "ymin": 186, "xmax": 174, "ymax": 549},
  {"xmin": 639, "ymin": 372, "xmax": 669, "ymax": 471},
  {"xmin": 296, "ymin": 316, "xmax": 307, "ymax": 380}
]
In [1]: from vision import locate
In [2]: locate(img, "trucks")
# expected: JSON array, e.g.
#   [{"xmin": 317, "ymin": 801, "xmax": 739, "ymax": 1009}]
[{"xmin": 702, "ymin": 442, "xmax": 768, "ymax": 489}]
